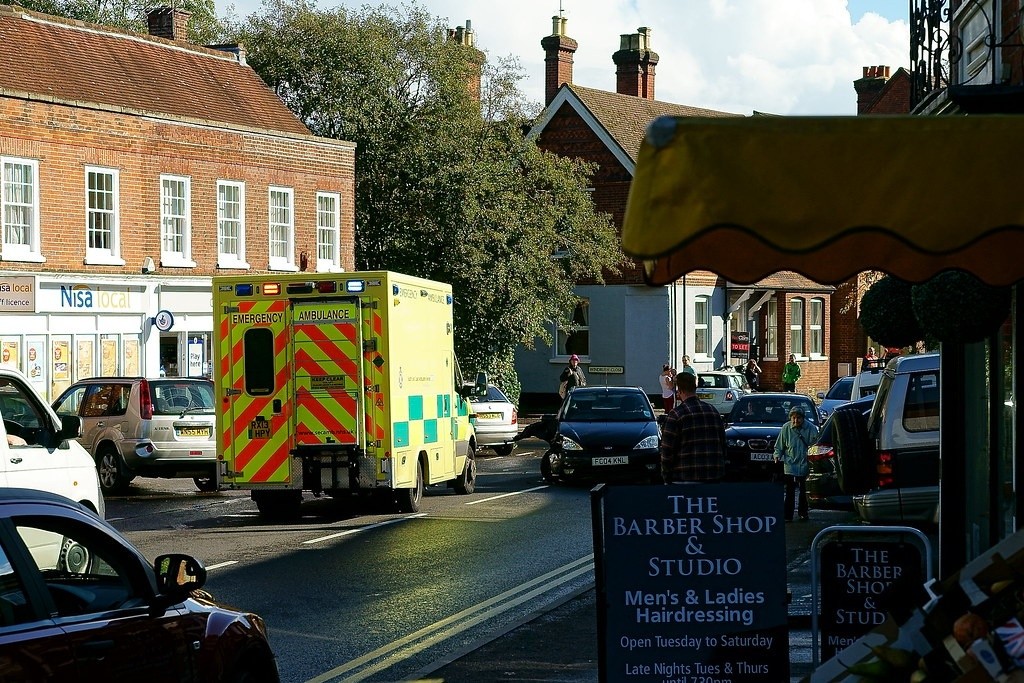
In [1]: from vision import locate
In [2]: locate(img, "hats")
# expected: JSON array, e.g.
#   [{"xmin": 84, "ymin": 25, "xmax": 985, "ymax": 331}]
[{"xmin": 569, "ymin": 354, "xmax": 580, "ymax": 362}]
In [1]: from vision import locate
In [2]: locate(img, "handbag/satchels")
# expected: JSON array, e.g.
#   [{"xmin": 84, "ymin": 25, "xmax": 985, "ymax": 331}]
[{"xmin": 559, "ymin": 381, "xmax": 568, "ymax": 399}]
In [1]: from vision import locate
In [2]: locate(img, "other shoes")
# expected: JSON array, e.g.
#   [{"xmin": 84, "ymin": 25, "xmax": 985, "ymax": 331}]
[{"xmin": 786, "ymin": 515, "xmax": 809, "ymax": 522}]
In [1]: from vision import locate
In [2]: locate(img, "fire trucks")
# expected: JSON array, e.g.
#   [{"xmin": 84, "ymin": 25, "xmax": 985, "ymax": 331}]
[{"xmin": 211, "ymin": 269, "xmax": 490, "ymax": 517}]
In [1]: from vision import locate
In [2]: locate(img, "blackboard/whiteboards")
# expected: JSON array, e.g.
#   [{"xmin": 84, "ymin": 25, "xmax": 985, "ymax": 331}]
[
  {"xmin": 588, "ymin": 474, "xmax": 804, "ymax": 683},
  {"xmin": 810, "ymin": 524, "xmax": 933, "ymax": 669}
]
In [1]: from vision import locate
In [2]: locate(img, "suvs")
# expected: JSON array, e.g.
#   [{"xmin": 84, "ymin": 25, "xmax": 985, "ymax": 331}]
[
  {"xmin": 26, "ymin": 376, "xmax": 222, "ymax": 493},
  {"xmin": 831, "ymin": 353, "xmax": 942, "ymax": 531}
]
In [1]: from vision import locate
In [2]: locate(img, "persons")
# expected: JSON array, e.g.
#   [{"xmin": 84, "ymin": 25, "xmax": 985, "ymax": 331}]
[
  {"xmin": 682, "ymin": 355, "xmax": 699, "ymax": 390},
  {"xmin": 659, "ymin": 364, "xmax": 675, "ymax": 414},
  {"xmin": 660, "ymin": 373, "xmax": 729, "ymax": 485},
  {"xmin": 881, "ymin": 346, "xmax": 903, "ymax": 369},
  {"xmin": 781, "ymin": 354, "xmax": 801, "ymax": 393},
  {"xmin": 773, "ymin": 405, "xmax": 820, "ymax": 523},
  {"xmin": 745, "ymin": 360, "xmax": 762, "ymax": 392},
  {"xmin": 863, "ymin": 346, "xmax": 880, "ymax": 370},
  {"xmin": 559, "ymin": 354, "xmax": 586, "ymax": 400}
]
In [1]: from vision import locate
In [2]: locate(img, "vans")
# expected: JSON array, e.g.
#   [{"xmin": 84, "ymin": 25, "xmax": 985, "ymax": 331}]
[{"xmin": 1, "ymin": 362, "xmax": 109, "ymax": 591}]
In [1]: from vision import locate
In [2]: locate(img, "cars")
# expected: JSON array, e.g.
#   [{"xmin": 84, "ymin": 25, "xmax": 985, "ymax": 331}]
[
  {"xmin": 544, "ymin": 388, "xmax": 664, "ymax": 487},
  {"xmin": 462, "ymin": 379, "xmax": 519, "ymax": 460},
  {"xmin": 0, "ymin": 484, "xmax": 280, "ymax": 683},
  {"xmin": 816, "ymin": 375, "xmax": 854, "ymax": 426},
  {"xmin": 720, "ymin": 389, "xmax": 828, "ymax": 476},
  {"xmin": 674, "ymin": 370, "xmax": 748, "ymax": 423}
]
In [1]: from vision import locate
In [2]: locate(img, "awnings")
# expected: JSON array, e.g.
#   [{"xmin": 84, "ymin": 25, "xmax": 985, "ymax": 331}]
[{"xmin": 617, "ymin": 111, "xmax": 1024, "ymax": 291}]
